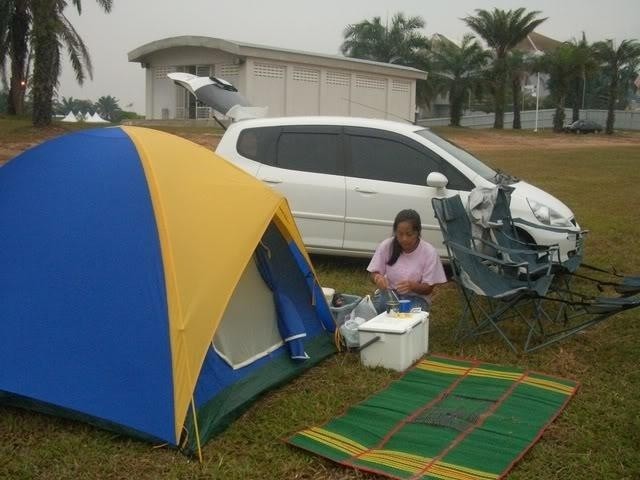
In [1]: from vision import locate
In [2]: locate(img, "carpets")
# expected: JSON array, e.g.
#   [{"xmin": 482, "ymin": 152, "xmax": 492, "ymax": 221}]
[{"xmin": 285, "ymin": 352, "xmax": 580, "ymax": 480}]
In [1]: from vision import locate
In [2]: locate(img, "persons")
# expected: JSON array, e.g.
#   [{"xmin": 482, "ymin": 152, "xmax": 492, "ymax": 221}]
[{"xmin": 366, "ymin": 209, "xmax": 448, "ymax": 315}]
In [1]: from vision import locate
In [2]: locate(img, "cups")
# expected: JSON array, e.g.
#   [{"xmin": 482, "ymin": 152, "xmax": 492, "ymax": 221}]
[
  {"xmin": 399, "ymin": 300, "xmax": 410, "ymax": 313},
  {"xmin": 385, "ymin": 301, "xmax": 398, "ymax": 317}
]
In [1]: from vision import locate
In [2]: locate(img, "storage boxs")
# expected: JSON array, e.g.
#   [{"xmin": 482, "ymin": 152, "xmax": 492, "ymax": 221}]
[
  {"xmin": 326, "ymin": 292, "xmax": 363, "ymax": 329},
  {"xmin": 358, "ymin": 307, "xmax": 430, "ymax": 374}
]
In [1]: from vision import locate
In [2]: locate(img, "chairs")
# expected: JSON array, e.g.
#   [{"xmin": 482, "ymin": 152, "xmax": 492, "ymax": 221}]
[
  {"xmin": 469, "ymin": 183, "xmax": 639, "ymax": 328},
  {"xmin": 431, "ymin": 194, "xmax": 640, "ymax": 361}
]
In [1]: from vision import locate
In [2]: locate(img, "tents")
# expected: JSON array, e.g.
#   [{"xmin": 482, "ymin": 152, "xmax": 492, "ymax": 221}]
[{"xmin": 0, "ymin": 124, "xmax": 350, "ymax": 464}]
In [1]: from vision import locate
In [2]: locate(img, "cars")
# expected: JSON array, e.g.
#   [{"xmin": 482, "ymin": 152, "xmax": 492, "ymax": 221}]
[
  {"xmin": 562, "ymin": 119, "xmax": 603, "ymax": 134},
  {"xmin": 166, "ymin": 72, "xmax": 581, "ymax": 265}
]
